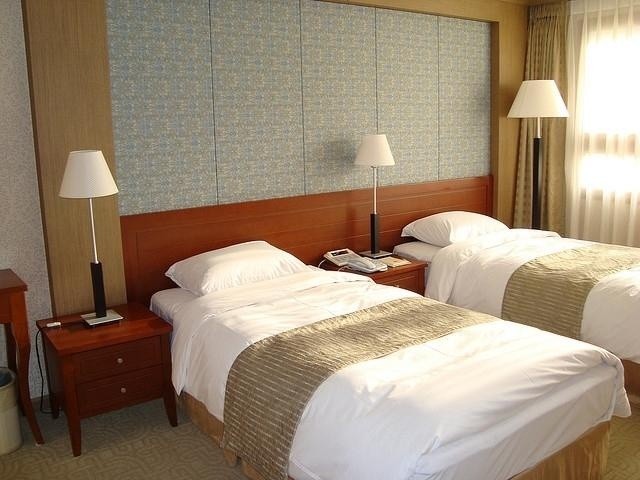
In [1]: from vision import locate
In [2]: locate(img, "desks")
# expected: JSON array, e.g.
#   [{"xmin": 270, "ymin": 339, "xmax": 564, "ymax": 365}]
[{"xmin": 0, "ymin": 268, "xmax": 45, "ymax": 446}]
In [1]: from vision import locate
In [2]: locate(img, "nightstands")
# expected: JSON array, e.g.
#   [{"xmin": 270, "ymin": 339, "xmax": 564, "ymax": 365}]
[
  {"xmin": 36, "ymin": 301, "xmax": 177, "ymax": 457},
  {"xmin": 318, "ymin": 247, "xmax": 428, "ymax": 298}
]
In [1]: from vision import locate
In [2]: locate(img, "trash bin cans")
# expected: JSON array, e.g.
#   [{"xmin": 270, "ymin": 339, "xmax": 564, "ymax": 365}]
[{"xmin": 0, "ymin": 366, "xmax": 27, "ymax": 456}]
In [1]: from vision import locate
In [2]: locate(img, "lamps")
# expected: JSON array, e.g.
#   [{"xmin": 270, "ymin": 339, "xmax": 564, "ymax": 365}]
[
  {"xmin": 59, "ymin": 149, "xmax": 123, "ymax": 318},
  {"xmin": 353, "ymin": 133, "xmax": 395, "ymax": 259},
  {"xmin": 507, "ymin": 79, "xmax": 569, "ymax": 230}
]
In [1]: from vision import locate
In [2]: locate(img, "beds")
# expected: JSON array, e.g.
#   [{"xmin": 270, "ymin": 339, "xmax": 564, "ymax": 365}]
[
  {"xmin": 150, "ymin": 263, "xmax": 631, "ymax": 480},
  {"xmin": 394, "ymin": 228, "xmax": 640, "ymax": 399}
]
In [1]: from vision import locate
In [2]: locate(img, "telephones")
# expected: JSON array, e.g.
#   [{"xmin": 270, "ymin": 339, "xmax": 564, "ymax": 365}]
[{"xmin": 348, "ymin": 256, "xmax": 388, "ymax": 274}]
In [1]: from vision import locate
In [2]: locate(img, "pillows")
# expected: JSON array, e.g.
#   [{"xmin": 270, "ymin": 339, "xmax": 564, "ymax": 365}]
[
  {"xmin": 165, "ymin": 241, "xmax": 313, "ymax": 298},
  {"xmin": 400, "ymin": 211, "xmax": 511, "ymax": 247}
]
